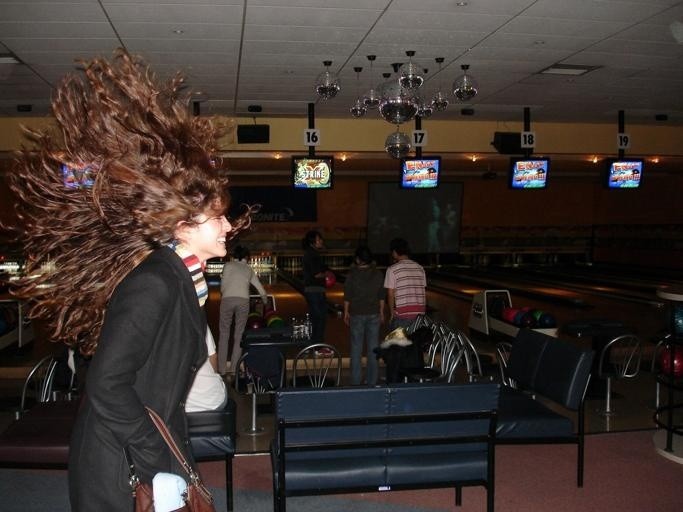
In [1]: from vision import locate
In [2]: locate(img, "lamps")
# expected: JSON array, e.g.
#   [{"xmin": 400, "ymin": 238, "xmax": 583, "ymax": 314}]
[{"xmin": 316, "ymin": 51, "xmax": 478, "ymax": 159}]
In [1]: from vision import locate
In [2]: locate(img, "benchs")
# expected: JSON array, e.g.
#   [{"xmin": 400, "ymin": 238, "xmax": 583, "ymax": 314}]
[
  {"xmin": 268, "ymin": 379, "xmax": 501, "ymax": 512},
  {"xmin": 497, "ymin": 331, "xmax": 596, "ymax": 488}
]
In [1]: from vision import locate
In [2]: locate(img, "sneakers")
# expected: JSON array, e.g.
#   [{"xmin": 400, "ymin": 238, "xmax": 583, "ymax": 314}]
[{"xmin": 315, "ymin": 348, "xmax": 334, "ymax": 356}]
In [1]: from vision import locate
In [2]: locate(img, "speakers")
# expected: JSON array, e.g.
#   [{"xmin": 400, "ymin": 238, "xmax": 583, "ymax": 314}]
[
  {"xmin": 236, "ymin": 123, "xmax": 270, "ymax": 146},
  {"xmin": 492, "ymin": 131, "xmax": 534, "ymax": 155}
]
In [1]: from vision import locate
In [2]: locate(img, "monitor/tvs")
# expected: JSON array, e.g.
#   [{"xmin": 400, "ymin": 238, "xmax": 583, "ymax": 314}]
[
  {"xmin": 400, "ymin": 155, "xmax": 441, "ymax": 191},
  {"xmin": 204, "ymin": 153, "xmax": 223, "ymax": 176},
  {"xmin": 602, "ymin": 157, "xmax": 642, "ymax": 190},
  {"xmin": 510, "ymin": 158, "xmax": 549, "ymax": 190},
  {"xmin": 61, "ymin": 158, "xmax": 98, "ymax": 192},
  {"xmin": 289, "ymin": 153, "xmax": 335, "ymax": 190}
]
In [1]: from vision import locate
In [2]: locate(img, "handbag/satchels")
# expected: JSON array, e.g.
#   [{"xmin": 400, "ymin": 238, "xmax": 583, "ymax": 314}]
[{"xmin": 130, "ymin": 472, "xmax": 216, "ymax": 512}]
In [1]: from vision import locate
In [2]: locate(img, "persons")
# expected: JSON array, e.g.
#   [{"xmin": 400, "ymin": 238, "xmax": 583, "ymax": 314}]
[
  {"xmin": 383, "ymin": 240, "xmax": 427, "ymax": 383},
  {"xmin": 342, "ymin": 246, "xmax": 387, "ymax": 386},
  {"xmin": 217, "ymin": 244, "xmax": 268, "ymax": 384},
  {"xmin": 1, "ymin": 49, "xmax": 233, "ymax": 512},
  {"xmin": 300, "ymin": 231, "xmax": 332, "ymax": 350}
]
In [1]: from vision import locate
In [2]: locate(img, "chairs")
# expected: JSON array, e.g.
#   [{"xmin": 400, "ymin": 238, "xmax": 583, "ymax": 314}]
[
  {"xmin": 236, "ymin": 314, "xmax": 519, "ymax": 435},
  {"xmin": 186, "ymin": 400, "xmax": 237, "ymax": 511},
  {"xmin": 597, "ymin": 332, "xmax": 644, "ymax": 418},
  {"xmin": 19, "ymin": 355, "xmax": 58, "ymax": 417},
  {"xmin": 647, "ymin": 333, "xmax": 671, "ymax": 412}
]
